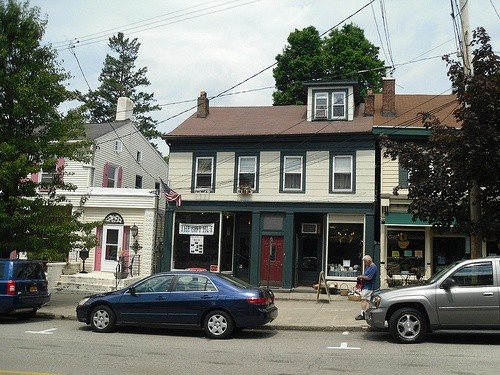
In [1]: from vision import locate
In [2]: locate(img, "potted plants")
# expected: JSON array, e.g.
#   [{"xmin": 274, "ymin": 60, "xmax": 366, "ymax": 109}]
[
  {"xmin": 241, "ymin": 185, "xmax": 251, "ymax": 194},
  {"xmin": 114, "ymin": 252, "xmax": 129, "ymax": 278}
]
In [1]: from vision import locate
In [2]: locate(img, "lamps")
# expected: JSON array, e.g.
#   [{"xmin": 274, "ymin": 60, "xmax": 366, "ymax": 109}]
[
  {"xmin": 131, "ymin": 222, "xmax": 139, "ymax": 243},
  {"xmin": 79, "ymin": 247, "xmax": 89, "ymax": 273}
]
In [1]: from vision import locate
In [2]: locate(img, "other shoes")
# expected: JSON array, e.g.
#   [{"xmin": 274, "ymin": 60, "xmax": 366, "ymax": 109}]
[{"xmin": 355, "ymin": 314, "xmax": 364, "ymax": 320}]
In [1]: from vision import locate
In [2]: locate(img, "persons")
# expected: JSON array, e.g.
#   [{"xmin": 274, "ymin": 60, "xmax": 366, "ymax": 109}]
[{"xmin": 355, "ymin": 255, "xmax": 380, "ymax": 320}]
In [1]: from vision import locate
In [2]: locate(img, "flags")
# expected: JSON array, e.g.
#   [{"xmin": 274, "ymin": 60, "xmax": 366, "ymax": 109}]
[{"xmin": 159, "ymin": 178, "xmax": 182, "ymax": 206}]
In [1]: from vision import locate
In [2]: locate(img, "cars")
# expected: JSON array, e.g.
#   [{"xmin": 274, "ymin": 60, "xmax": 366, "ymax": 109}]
[
  {"xmin": 356, "ymin": 258, "xmax": 500, "ymax": 344},
  {"xmin": 77, "ymin": 272, "xmax": 279, "ymax": 340}
]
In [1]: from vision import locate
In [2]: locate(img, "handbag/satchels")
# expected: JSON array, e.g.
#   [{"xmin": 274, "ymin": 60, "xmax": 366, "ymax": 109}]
[{"xmin": 354, "ymin": 279, "xmax": 365, "ymax": 291}]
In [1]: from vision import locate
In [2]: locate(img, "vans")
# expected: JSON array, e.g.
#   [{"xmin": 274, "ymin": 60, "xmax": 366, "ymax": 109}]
[{"xmin": 0, "ymin": 259, "xmax": 49, "ymax": 320}]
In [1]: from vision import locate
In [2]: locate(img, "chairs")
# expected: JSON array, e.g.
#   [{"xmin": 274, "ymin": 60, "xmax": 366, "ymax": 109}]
[
  {"xmin": 188, "ymin": 281, "xmax": 198, "ymax": 289},
  {"xmin": 175, "ymin": 279, "xmax": 191, "ymax": 293}
]
